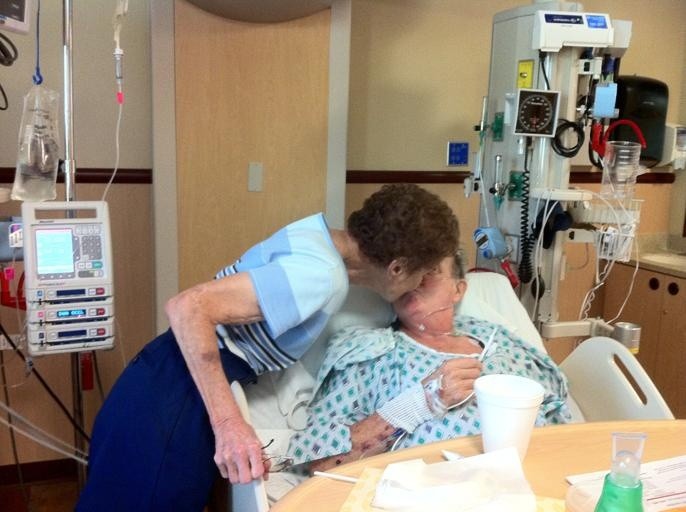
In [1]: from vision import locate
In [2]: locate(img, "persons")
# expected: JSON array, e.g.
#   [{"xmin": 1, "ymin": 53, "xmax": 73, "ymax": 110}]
[
  {"xmin": 75, "ymin": 184, "xmax": 461, "ymax": 512},
  {"xmin": 288, "ymin": 253, "xmax": 574, "ymax": 477}
]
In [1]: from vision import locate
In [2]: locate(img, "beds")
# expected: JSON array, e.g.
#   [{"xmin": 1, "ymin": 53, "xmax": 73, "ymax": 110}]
[{"xmin": 226, "ymin": 264, "xmax": 686, "ymax": 512}]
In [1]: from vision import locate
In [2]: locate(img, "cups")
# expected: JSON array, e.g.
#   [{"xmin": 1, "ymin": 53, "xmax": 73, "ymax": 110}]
[
  {"xmin": 600, "ymin": 141, "xmax": 641, "ymax": 202},
  {"xmin": 473, "ymin": 372, "xmax": 549, "ymax": 471}
]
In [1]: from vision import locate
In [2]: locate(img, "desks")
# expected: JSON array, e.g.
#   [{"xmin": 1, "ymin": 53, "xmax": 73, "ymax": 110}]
[{"xmin": 268, "ymin": 416, "xmax": 686, "ymax": 512}]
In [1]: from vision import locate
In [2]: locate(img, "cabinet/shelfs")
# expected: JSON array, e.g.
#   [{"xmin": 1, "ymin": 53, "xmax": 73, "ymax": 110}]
[{"xmin": 596, "ymin": 253, "xmax": 685, "ymax": 419}]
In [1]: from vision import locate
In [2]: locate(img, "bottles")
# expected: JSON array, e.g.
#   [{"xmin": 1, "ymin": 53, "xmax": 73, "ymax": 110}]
[{"xmin": 590, "ymin": 432, "xmax": 651, "ymax": 512}]
